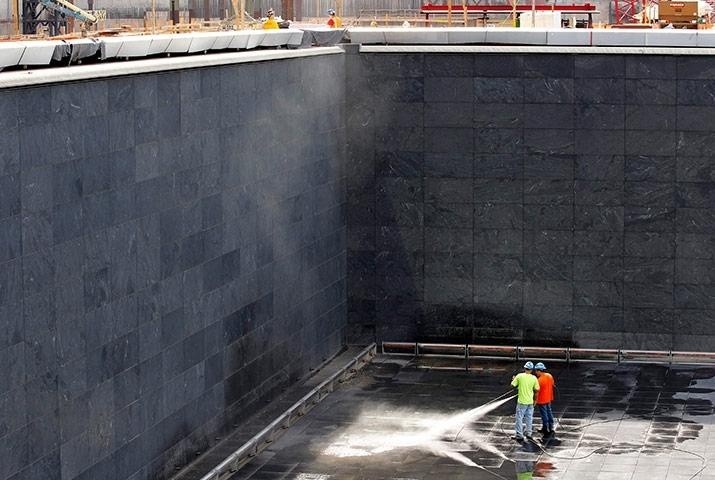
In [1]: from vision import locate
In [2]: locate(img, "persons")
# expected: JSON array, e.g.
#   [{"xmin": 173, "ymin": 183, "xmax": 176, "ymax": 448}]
[
  {"xmin": 534, "ymin": 362, "xmax": 554, "ymax": 433},
  {"xmin": 328, "ymin": 9, "xmax": 342, "ymax": 29},
  {"xmin": 511, "ymin": 361, "xmax": 540, "ymax": 442},
  {"xmin": 263, "ymin": 10, "xmax": 279, "ymax": 29}
]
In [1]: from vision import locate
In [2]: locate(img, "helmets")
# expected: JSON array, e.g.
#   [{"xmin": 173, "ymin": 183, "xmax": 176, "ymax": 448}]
[
  {"xmin": 524, "ymin": 361, "xmax": 534, "ymax": 370},
  {"xmin": 535, "ymin": 362, "xmax": 547, "ymax": 370}
]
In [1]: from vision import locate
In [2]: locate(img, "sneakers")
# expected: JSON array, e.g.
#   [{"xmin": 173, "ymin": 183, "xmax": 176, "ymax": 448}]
[{"xmin": 511, "ymin": 430, "xmax": 555, "ymax": 441}]
